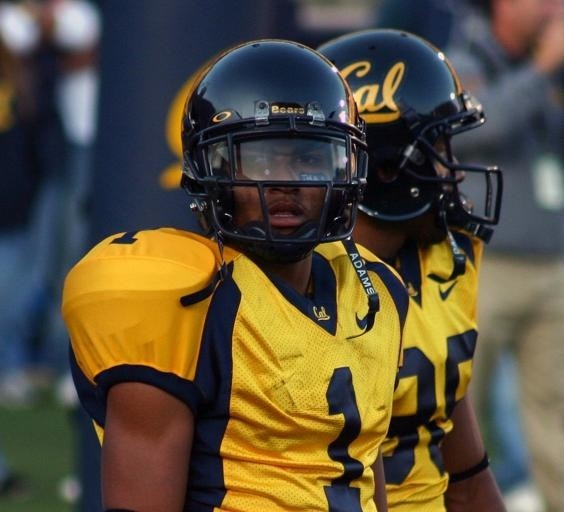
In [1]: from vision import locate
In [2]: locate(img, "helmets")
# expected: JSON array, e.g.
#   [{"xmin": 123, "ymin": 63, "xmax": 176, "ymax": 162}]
[
  {"xmin": 316, "ymin": 28, "xmax": 503, "ymax": 243},
  {"xmin": 182, "ymin": 39, "xmax": 369, "ymax": 266}
]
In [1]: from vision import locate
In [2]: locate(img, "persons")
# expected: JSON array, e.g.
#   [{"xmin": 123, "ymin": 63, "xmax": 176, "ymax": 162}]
[
  {"xmin": 315, "ymin": 27, "xmax": 510, "ymax": 511},
  {"xmin": 440, "ymin": 0, "xmax": 564, "ymax": 511},
  {"xmin": 62, "ymin": 40, "xmax": 408, "ymax": 511},
  {"xmin": 2, "ymin": 0, "xmax": 483, "ymax": 410}
]
